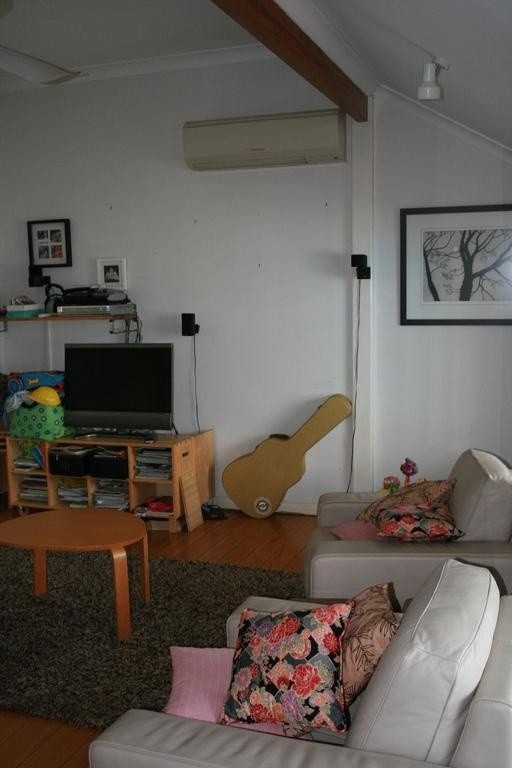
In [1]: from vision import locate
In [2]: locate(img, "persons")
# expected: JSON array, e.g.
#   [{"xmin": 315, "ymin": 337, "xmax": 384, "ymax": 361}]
[{"xmin": 105, "ymin": 266, "xmax": 119, "ymax": 282}]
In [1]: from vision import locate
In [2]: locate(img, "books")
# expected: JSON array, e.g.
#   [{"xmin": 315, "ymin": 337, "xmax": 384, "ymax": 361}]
[
  {"xmin": 18, "ymin": 477, "xmax": 48, "ymax": 502},
  {"xmin": 134, "ymin": 495, "xmax": 174, "ymax": 517},
  {"xmin": 55, "ymin": 477, "xmax": 88, "ymax": 508},
  {"xmin": 13, "ymin": 444, "xmax": 43, "ymax": 472},
  {"xmin": 50, "ymin": 444, "xmax": 92, "ymax": 452},
  {"xmin": 93, "ymin": 480, "xmax": 129, "ymax": 512},
  {"xmin": 93, "ymin": 447, "xmax": 128, "ymax": 457},
  {"xmin": 134, "ymin": 447, "xmax": 173, "ymax": 480}
]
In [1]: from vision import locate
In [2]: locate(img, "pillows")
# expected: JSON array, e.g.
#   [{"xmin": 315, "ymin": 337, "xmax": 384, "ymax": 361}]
[
  {"xmin": 216, "ymin": 600, "xmax": 356, "ymax": 734},
  {"xmin": 355, "ymin": 478, "xmax": 458, "ymax": 522},
  {"xmin": 165, "ymin": 646, "xmax": 285, "ymax": 736},
  {"xmin": 366, "ymin": 502, "xmax": 466, "ymax": 543},
  {"xmin": 343, "ymin": 582, "xmax": 400, "ymax": 708},
  {"xmin": 329, "ymin": 520, "xmax": 377, "ymax": 540}
]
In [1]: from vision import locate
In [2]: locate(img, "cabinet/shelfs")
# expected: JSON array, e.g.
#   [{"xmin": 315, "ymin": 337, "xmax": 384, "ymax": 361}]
[{"xmin": 5, "ymin": 428, "xmax": 216, "ymax": 533}]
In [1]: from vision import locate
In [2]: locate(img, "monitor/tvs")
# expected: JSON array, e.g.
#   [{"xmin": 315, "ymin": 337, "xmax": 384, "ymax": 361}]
[{"xmin": 63, "ymin": 343, "xmax": 173, "ymax": 441}]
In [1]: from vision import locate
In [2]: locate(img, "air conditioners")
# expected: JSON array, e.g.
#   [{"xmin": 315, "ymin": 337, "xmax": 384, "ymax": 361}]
[{"xmin": 183, "ymin": 110, "xmax": 346, "ymax": 171}]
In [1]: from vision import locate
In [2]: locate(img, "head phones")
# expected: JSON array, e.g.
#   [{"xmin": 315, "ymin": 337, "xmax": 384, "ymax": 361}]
[{"xmin": 44, "ymin": 283, "xmax": 66, "ymax": 313}]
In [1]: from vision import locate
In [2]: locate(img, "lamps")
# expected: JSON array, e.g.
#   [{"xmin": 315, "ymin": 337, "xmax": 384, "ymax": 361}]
[{"xmin": 417, "ymin": 61, "xmax": 442, "ymax": 101}]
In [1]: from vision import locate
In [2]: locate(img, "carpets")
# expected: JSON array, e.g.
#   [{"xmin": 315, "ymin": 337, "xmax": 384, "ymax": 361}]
[{"xmin": 1, "ymin": 546, "xmax": 302, "ymax": 733}]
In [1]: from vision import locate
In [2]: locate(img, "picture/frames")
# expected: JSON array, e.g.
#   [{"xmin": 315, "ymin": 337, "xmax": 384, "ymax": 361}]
[
  {"xmin": 26, "ymin": 218, "xmax": 72, "ymax": 267},
  {"xmin": 97, "ymin": 257, "xmax": 127, "ymax": 289},
  {"xmin": 400, "ymin": 203, "xmax": 512, "ymax": 326}
]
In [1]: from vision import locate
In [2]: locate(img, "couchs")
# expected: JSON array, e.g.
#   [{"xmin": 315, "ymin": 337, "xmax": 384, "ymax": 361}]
[
  {"xmin": 309, "ymin": 447, "xmax": 511, "ymax": 597},
  {"xmin": 87, "ymin": 559, "xmax": 512, "ymax": 768}
]
